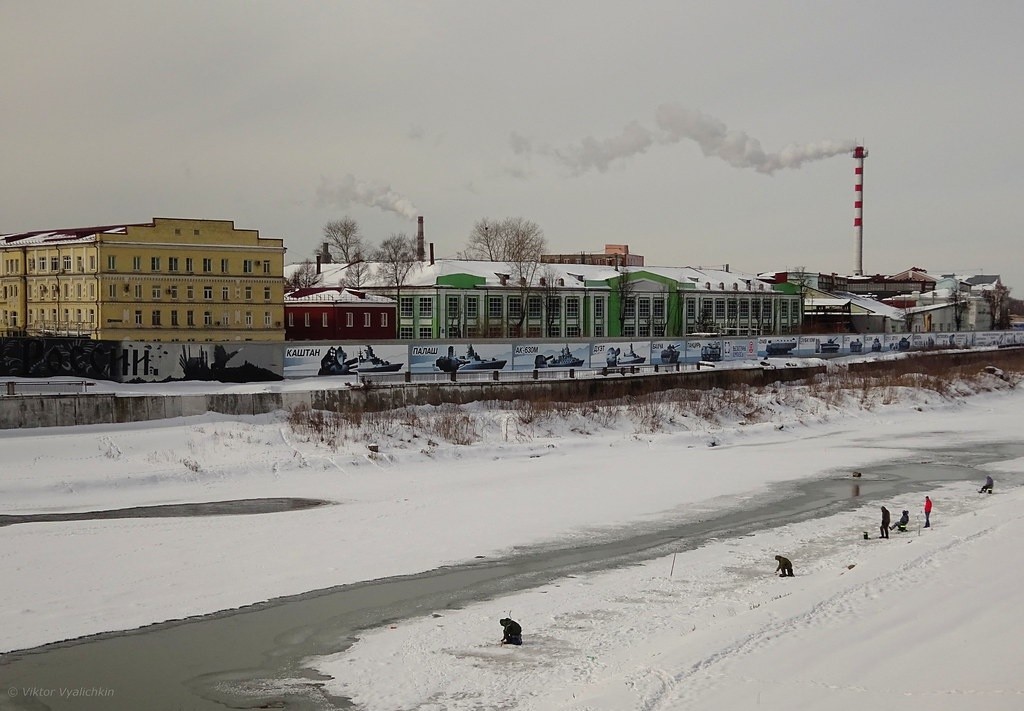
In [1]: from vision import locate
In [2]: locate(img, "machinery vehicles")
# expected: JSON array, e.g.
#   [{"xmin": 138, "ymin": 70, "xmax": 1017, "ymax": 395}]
[
  {"xmin": 820, "ymin": 337, "xmax": 840, "ymax": 353},
  {"xmin": 766, "ymin": 338, "xmax": 797, "ymax": 355},
  {"xmin": 872, "ymin": 338, "xmax": 881, "ymax": 350},
  {"xmin": 849, "ymin": 339, "xmax": 862, "ymax": 352},
  {"xmin": 701, "ymin": 341, "xmax": 721, "ymax": 362},
  {"xmin": 899, "ymin": 335, "xmax": 911, "ymax": 350}
]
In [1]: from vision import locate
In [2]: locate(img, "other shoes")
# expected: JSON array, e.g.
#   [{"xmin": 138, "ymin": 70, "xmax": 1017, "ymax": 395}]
[{"xmin": 889, "ymin": 526, "xmax": 892, "ymax": 530}]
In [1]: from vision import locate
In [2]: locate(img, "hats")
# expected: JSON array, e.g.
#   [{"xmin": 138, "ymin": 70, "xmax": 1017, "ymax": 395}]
[{"xmin": 500, "ymin": 619, "xmax": 506, "ymax": 626}]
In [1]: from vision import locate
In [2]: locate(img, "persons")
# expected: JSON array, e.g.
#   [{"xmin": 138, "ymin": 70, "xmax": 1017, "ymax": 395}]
[
  {"xmin": 879, "ymin": 506, "xmax": 890, "ymax": 539},
  {"xmin": 775, "ymin": 555, "xmax": 794, "ymax": 577},
  {"xmin": 923, "ymin": 496, "xmax": 932, "ymax": 528},
  {"xmin": 500, "ymin": 618, "xmax": 523, "ymax": 645},
  {"xmin": 889, "ymin": 510, "xmax": 909, "ymax": 530},
  {"xmin": 978, "ymin": 476, "xmax": 993, "ymax": 493}
]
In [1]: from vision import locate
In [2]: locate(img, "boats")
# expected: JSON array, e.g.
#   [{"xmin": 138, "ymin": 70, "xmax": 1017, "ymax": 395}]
[
  {"xmin": 548, "ymin": 344, "xmax": 585, "ymax": 367},
  {"xmin": 661, "ymin": 344, "xmax": 681, "ymax": 363},
  {"xmin": 617, "ymin": 343, "xmax": 646, "ymax": 364},
  {"xmin": 458, "ymin": 344, "xmax": 508, "ymax": 369},
  {"xmin": 348, "ymin": 345, "xmax": 404, "ymax": 373}
]
